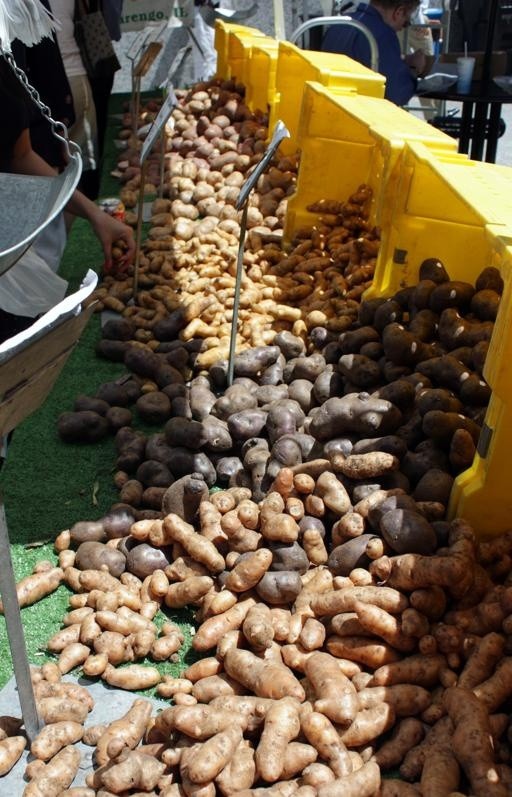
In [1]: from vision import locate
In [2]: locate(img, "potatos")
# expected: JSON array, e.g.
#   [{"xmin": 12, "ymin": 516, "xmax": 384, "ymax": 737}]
[{"xmin": 0, "ymin": 79, "xmax": 511, "ymax": 796}]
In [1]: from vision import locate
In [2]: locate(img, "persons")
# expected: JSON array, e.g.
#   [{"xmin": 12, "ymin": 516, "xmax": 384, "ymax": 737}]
[{"xmin": 319, "ymin": 0, "xmax": 427, "ymax": 106}]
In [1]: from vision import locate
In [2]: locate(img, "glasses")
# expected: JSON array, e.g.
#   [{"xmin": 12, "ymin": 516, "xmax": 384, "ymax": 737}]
[{"xmin": 404, "ymin": 12, "xmax": 412, "ymax": 29}]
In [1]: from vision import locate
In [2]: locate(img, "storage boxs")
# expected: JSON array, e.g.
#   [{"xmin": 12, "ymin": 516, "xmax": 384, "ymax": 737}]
[
  {"xmin": 363, "ymin": 140, "xmax": 512, "ymax": 535},
  {"xmin": 269, "ymin": 39, "xmax": 386, "ymax": 167},
  {"xmin": 213, "ymin": 18, "xmax": 277, "ymax": 114},
  {"xmin": 277, "ymin": 81, "xmax": 462, "ymax": 254}
]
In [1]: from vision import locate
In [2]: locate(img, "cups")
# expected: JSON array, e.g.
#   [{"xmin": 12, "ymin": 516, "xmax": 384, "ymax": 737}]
[{"xmin": 455, "ymin": 56, "xmax": 475, "ymax": 94}]
